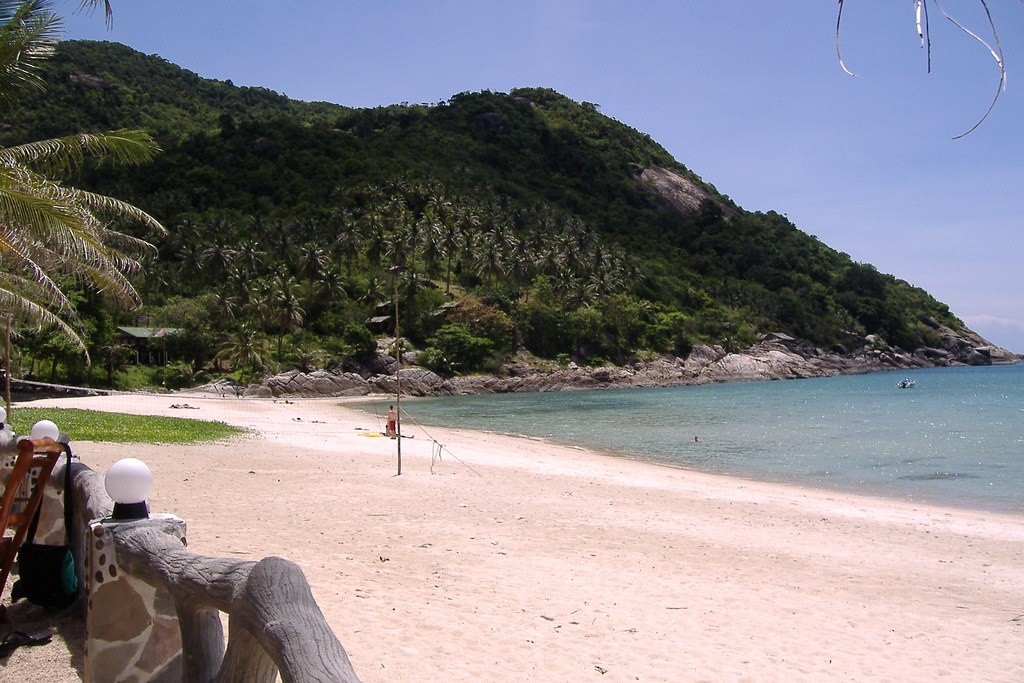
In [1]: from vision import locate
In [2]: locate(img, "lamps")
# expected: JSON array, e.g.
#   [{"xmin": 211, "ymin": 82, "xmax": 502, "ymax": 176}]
[
  {"xmin": 104, "ymin": 458, "xmax": 152, "ymax": 520},
  {"xmin": 0, "ymin": 406, "xmax": 7, "ymax": 429},
  {"xmin": 31, "ymin": 420, "xmax": 59, "ymax": 442}
]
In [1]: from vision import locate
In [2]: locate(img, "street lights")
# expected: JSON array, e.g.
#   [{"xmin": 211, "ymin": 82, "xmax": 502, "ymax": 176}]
[{"xmin": 386, "ymin": 265, "xmax": 408, "ymax": 472}]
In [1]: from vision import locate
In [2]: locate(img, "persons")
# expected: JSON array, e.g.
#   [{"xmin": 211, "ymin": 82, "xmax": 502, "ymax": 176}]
[
  {"xmin": 162, "ymin": 381, "xmax": 165, "ymax": 387},
  {"xmin": 223, "ymin": 393, "xmax": 225, "ymax": 398},
  {"xmin": 388, "ymin": 405, "xmax": 397, "ymax": 439},
  {"xmin": 237, "ymin": 391, "xmax": 240, "ymax": 399},
  {"xmin": 168, "ymin": 404, "xmax": 200, "ymax": 409},
  {"xmin": 694, "ymin": 437, "xmax": 698, "ymax": 442},
  {"xmin": 386, "ymin": 425, "xmax": 414, "ymax": 439},
  {"xmin": 409, "ymin": 393, "xmax": 411, "ymax": 396}
]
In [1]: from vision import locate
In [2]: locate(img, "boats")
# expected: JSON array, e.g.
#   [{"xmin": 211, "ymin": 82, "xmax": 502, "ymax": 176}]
[{"xmin": 896, "ymin": 378, "xmax": 916, "ymax": 389}]
